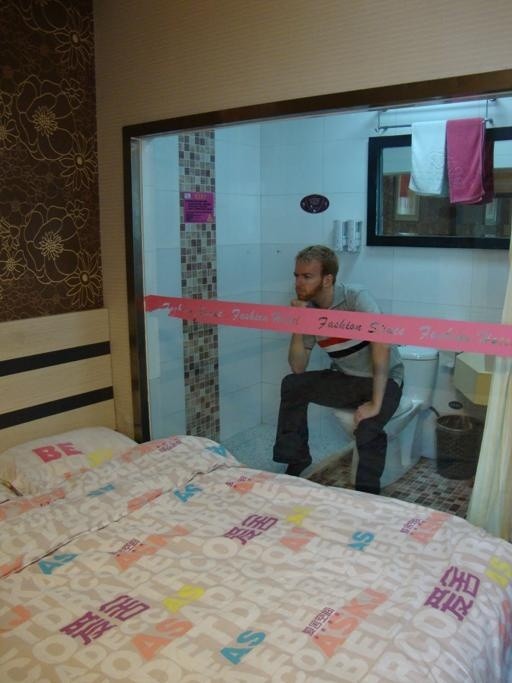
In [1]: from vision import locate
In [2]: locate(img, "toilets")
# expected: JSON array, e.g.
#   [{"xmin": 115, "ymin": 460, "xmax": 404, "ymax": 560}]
[{"xmin": 334, "ymin": 346, "xmax": 439, "ymax": 490}]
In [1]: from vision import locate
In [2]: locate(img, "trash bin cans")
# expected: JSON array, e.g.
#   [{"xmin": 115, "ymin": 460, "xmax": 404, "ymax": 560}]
[{"xmin": 437, "ymin": 414, "xmax": 484, "ymax": 479}]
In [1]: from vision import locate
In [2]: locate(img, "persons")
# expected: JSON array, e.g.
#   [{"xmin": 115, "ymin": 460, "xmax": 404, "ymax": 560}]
[{"xmin": 270, "ymin": 243, "xmax": 406, "ymax": 494}]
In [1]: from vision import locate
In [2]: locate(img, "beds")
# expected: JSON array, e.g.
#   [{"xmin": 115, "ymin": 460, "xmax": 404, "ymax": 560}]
[{"xmin": 0, "ymin": 307, "xmax": 512, "ymax": 682}]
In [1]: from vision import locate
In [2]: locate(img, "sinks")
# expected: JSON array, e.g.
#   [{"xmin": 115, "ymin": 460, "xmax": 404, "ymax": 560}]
[{"xmin": 455, "ymin": 351, "xmax": 496, "ymax": 373}]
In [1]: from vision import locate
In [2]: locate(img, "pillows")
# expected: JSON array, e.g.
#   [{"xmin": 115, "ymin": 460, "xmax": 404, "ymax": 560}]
[{"xmin": 1, "ymin": 423, "xmax": 140, "ymax": 496}]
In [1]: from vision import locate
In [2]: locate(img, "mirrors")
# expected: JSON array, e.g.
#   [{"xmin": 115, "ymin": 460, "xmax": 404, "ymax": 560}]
[{"xmin": 363, "ymin": 121, "xmax": 511, "ymax": 251}]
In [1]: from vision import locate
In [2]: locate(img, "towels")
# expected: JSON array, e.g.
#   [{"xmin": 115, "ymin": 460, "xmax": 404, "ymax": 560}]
[
  {"xmin": 442, "ymin": 112, "xmax": 487, "ymax": 202},
  {"xmin": 406, "ymin": 117, "xmax": 448, "ymax": 198}
]
